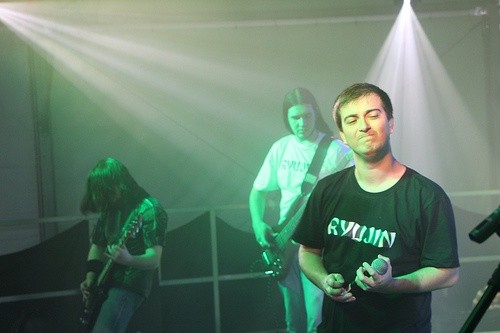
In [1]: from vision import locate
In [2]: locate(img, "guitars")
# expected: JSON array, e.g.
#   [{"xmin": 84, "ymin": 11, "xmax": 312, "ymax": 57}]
[
  {"xmin": 78, "ymin": 212, "xmax": 144, "ymax": 331},
  {"xmin": 253, "ymin": 152, "xmax": 352, "ymax": 280}
]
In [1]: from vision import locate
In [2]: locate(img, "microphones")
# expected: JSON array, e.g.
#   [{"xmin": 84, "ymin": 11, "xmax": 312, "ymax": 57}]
[{"xmin": 345, "ymin": 258, "xmax": 388, "ymax": 297}]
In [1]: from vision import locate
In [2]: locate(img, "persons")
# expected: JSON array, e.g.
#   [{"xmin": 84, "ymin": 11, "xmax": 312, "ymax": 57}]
[
  {"xmin": 250, "ymin": 88, "xmax": 356, "ymax": 333},
  {"xmin": 79, "ymin": 157, "xmax": 169, "ymax": 333},
  {"xmin": 291, "ymin": 83, "xmax": 460, "ymax": 333}
]
